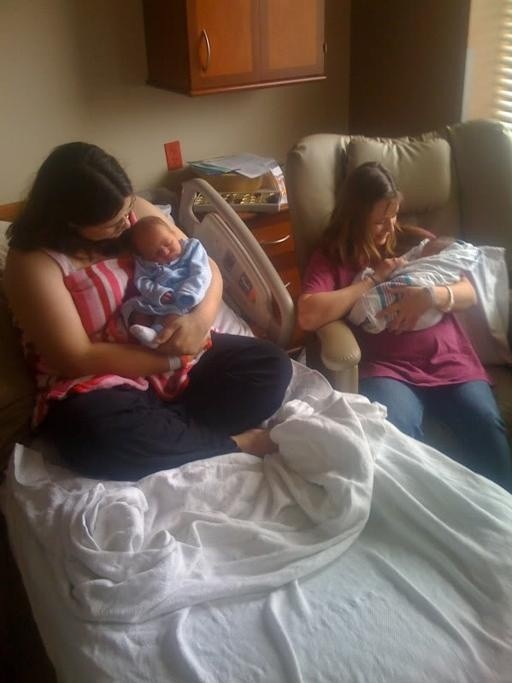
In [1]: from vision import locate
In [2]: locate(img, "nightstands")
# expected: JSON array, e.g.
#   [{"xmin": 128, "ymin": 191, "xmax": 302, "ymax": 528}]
[{"xmin": 194, "ymin": 205, "xmax": 309, "ymax": 355}]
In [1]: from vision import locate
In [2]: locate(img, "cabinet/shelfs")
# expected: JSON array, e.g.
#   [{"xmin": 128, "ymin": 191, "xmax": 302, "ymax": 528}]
[{"xmin": 142, "ymin": 0, "xmax": 328, "ymax": 97}]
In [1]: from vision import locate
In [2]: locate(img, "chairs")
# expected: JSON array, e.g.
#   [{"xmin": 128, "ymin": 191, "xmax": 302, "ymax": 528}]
[{"xmin": 284, "ymin": 118, "xmax": 512, "ymax": 459}]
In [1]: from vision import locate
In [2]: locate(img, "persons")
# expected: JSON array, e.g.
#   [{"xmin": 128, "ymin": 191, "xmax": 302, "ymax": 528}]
[
  {"xmin": 296, "ymin": 161, "xmax": 512, "ymax": 495},
  {"xmin": 119, "ymin": 214, "xmax": 211, "ymax": 350},
  {"xmin": 421, "ymin": 237, "xmax": 459, "ymax": 258},
  {"xmin": 0, "ymin": 141, "xmax": 293, "ymax": 482}
]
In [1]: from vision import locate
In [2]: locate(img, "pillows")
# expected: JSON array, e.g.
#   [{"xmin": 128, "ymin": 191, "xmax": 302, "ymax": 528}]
[
  {"xmin": 0, "ymin": 194, "xmax": 175, "ymax": 275},
  {"xmin": 346, "ymin": 134, "xmax": 454, "ymax": 218}
]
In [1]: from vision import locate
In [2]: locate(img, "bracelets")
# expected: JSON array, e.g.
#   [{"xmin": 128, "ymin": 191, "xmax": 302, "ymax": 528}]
[
  {"xmin": 424, "ymin": 285, "xmax": 437, "ymax": 309},
  {"xmin": 442, "ymin": 284, "xmax": 455, "ymax": 315},
  {"xmin": 166, "ymin": 353, "xmax": 182, "ymax": 371},
  {"xmin": 368, "ymin": 274, "xmax": 380, "ymax": 286}
]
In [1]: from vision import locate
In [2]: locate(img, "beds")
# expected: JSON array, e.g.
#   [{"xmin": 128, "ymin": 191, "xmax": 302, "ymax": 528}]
[{"xmin": 0, "ymin": 176, "xmax": 512, "ymax": 682}]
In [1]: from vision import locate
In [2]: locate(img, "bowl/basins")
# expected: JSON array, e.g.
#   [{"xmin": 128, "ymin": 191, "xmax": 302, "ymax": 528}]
[{"xmin": 189, "ymin": 166, "xmax": 264, "ymax": 193}]
